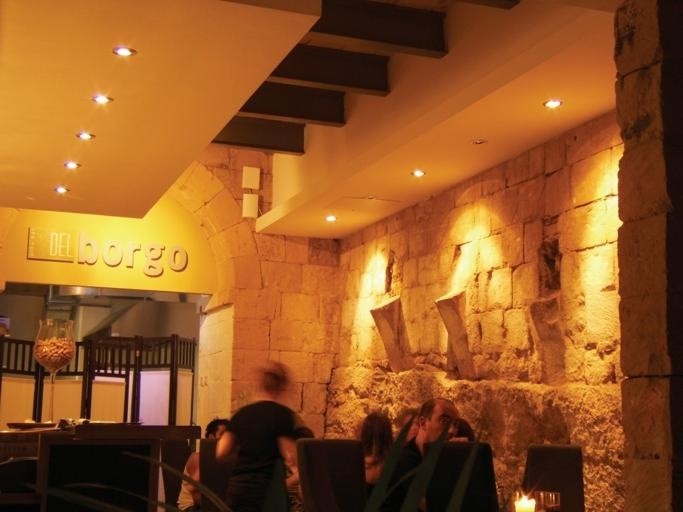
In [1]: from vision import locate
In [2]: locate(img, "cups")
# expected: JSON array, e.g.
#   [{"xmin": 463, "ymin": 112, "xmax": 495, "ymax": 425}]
[{"xmin": 535, "ymin": 491, "xmax": 561, "ymax": 511}]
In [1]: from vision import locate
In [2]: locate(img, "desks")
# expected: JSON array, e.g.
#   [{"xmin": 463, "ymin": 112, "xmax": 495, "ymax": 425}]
[{"xmin": 0, "ymin": 425, "xmax": 201, "ymax": 512}]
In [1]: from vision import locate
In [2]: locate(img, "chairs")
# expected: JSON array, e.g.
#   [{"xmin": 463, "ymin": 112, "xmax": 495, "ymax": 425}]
[
  {"xmin": 37, "ymin": 430, "xmax": 160, "ymax": 512},
  {"xmin": 518, "ymin": 443, "xmax": 585, "ymax": 512},
  {"xmin": 162, "ymin": 440, "xmax": 191, "ymax": 512},
  {"xmin": 295, "ymin": 438, "xmax": 367, "ymax": 512},
  {"xmin": 423, "ymin": 440, "xmax": 500, "ymax": 511},
  {"xmin": 198, "ymin": 437, "xmax": 233, "ymax": 512}
]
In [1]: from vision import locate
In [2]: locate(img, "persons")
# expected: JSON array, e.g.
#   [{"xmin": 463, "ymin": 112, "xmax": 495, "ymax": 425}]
[
  {"xmin": 178, "ymin": 361, "xmax": 316, "ymax": 512},
  {"xmin": 357, "ymin": 397, "xmax": 475, "ymax": 512}
]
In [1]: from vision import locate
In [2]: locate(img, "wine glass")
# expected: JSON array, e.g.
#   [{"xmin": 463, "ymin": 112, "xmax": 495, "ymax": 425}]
[{"xmin": 31, "ymin": 317, "xmax": 75, "ymax": 421}]
